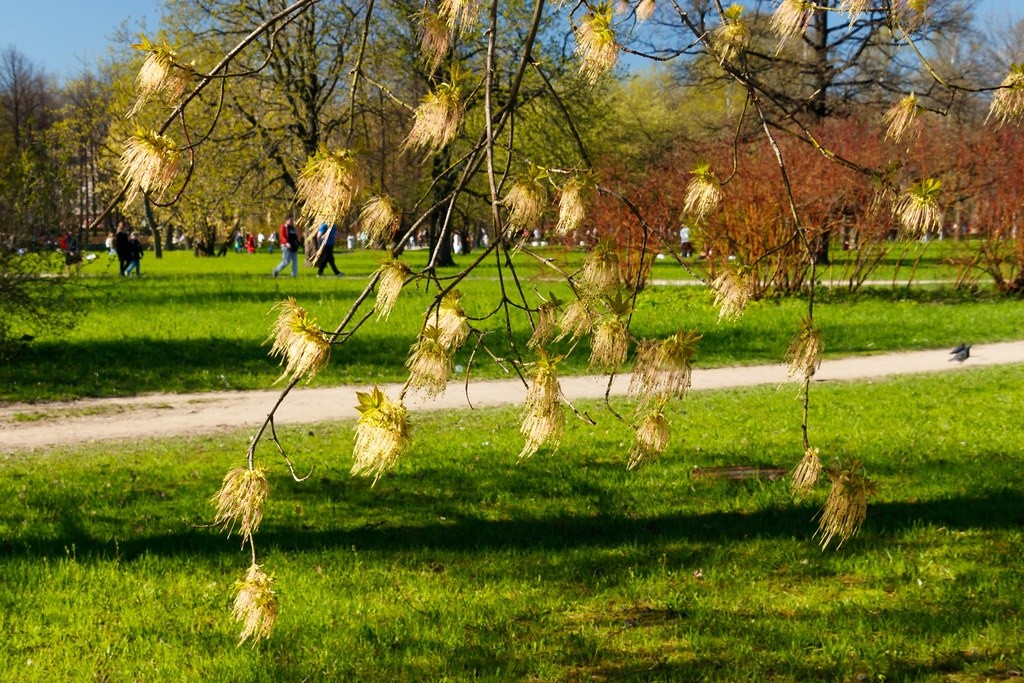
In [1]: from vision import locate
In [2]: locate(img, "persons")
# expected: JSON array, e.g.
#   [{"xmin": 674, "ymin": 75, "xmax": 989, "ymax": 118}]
[
  {"xmin": 63, "ymin": 230, "xmax": 86, "ymax": 278},
  {"xmin": 317, "ymin": 217, "xmax": 345, "ymax": 279},
  {"xmin": 24, "ymin": 211, "xmax": 904, "ymax": 262},
  {"xmin": 124, "ymin": 232, "xmax": 144, "ymax": 276},
  {"xmin": 272, "ymin": 215, "xmax": 300, "ymax": 278},
  {"xmin": 114, "ymin": 221, "xmax": 133, "ymax": 276}
]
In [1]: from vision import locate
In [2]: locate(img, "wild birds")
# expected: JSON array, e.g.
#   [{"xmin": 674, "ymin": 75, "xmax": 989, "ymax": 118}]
[{"xmin": 949, "ymin": 342, "xmax": 972, "ymax": 364}]
